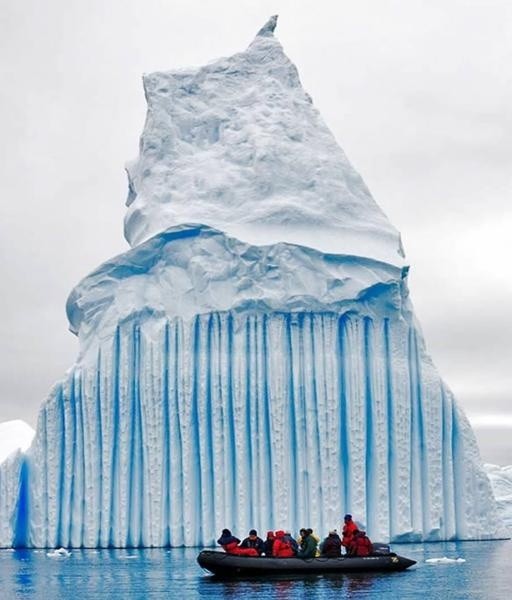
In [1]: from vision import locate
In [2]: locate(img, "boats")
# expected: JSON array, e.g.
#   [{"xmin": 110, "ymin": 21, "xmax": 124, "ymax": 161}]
[{"xmin": 197, "ymin": 550, "xmax": 417, "ymax": 579}]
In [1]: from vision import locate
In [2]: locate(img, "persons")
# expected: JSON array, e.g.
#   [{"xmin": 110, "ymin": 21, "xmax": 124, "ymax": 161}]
[{"xmin": 217, "ymin": 514, "xmax": 373, "ymax": 558}]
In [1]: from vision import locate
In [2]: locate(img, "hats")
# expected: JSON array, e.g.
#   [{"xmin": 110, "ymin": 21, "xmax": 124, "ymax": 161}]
[{"xmin": 222, "ymin": 514, "xmax": 360, "ymax": 538}]
